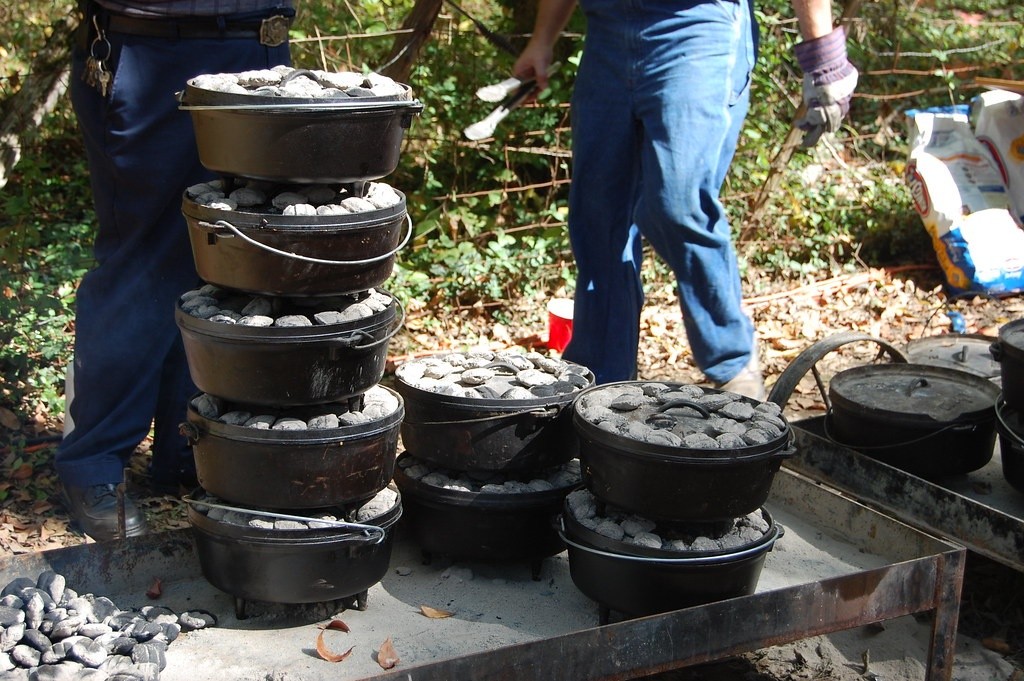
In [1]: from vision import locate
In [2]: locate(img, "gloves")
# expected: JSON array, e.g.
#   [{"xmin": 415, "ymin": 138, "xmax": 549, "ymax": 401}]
[{"xmin": 795, "ymin": 24, "xmax": 860, "ymax": 149}]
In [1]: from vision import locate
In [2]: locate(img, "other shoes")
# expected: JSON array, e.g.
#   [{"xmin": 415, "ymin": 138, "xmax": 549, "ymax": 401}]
[{"xmin": 715, "ymin": 317, "xmax": 767, "ymax": 401}]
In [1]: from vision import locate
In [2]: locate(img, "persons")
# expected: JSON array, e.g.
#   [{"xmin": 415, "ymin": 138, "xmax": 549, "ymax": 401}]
[
  {"xmin": 511, "ymin": 0, "xmax": 857, "ymax": 401},
  {"xmin": 61, "ymin": 0, "xmax": 300, "ymax": 541}
]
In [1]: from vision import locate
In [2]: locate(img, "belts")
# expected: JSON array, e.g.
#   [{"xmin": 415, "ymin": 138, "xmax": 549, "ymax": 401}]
[{"xmin": 79, "ymin": 2, "xmax": 296, "ymax": 37}]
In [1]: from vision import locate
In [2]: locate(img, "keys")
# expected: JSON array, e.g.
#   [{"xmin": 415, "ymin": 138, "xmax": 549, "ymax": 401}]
[{"xmin": 84, "ymin": 55, "xmax": 115, "ymax": 96}]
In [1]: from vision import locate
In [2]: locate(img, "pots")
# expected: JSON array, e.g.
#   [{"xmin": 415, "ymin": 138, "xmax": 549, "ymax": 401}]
[
  {"xmin": 176, "ymin": 69, "xmax": 796, "ymax": 633},
  {"xmin": 825, "ymin": 317, "xmax": 1024, "ymax": 502}
]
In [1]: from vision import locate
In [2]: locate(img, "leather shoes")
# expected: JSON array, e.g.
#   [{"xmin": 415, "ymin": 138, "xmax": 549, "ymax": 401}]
[
  {"xmin": 59, "ymin": 475, "xmax": 149, "ymax": 543},
  {"xmin": 143, "ymin": 461, "xmax": 198, "ymax": 491}
]
